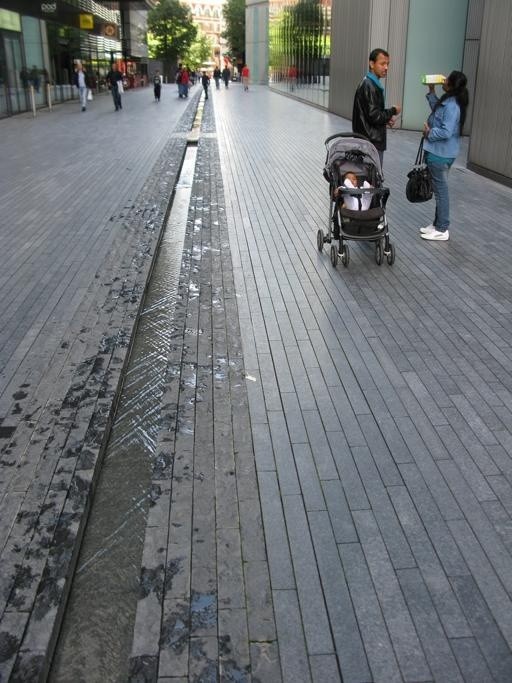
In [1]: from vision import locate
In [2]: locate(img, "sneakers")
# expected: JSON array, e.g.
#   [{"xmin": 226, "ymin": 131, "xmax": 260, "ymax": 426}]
[
  {"xmin": 114, "ymin": 105, "xmax": 123, "ymax": 112},
  {"xmin": 82, "ymin": 107, "xmax": 86, "ymax": 111},
  {"xmin": 420, "ymin": 223, "xmax": 449, "ymax": 241}
]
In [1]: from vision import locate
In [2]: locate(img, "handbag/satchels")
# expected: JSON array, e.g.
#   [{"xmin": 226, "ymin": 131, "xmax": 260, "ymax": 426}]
[
  {"xmin": 87, "ymin": 89, "xmax": 93, "ymax": 101},
  {"xmin": 406, "ymin": 167, "xmax": 433, "ymax": 203}
]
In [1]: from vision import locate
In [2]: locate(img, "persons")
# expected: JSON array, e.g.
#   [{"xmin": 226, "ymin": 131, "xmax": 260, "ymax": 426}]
[
  {"xmin": 19, "ymin": 65, "xmax": 30, "ymax": 96},
  {"xmin": 288, "ymin": 64, "xmax": 297, "ymax": 91},
  {"xmin": 174, "ymin": 62, "xmax": 231, "ymax": 99},
  {"xmin": 153, "ymin": 69, "xmax": 162, "ymax": 103},
  {"xmin": 72, "ymin": 60, "xmax": 93, "ymax": 111},
  {"xmin": 418, "ymin": 69, "xmax": 470, "ymax": 241},
  {"xmin": 30, "ymin": 65, "xmax": 44, "ymax": 93},
  {"xmin": 105, "ymin": 62, "xmax": 123, "ymax": 110},
  {"xmin": 241, "ymin": 63, "xmax": 250, "ymax": 91},
  {"xmin": 334, "ymin": 171, "xmax": 375, "ymax": 211},
  {"xmin": 352, "ymin": 47, "xmax": 402, "ymax": 169}
]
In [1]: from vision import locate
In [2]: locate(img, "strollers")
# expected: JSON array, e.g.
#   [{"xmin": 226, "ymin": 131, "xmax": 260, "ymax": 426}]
[{"xmin": 314, "ymin": 129, "xmax": 396, "ymax": 270}]
